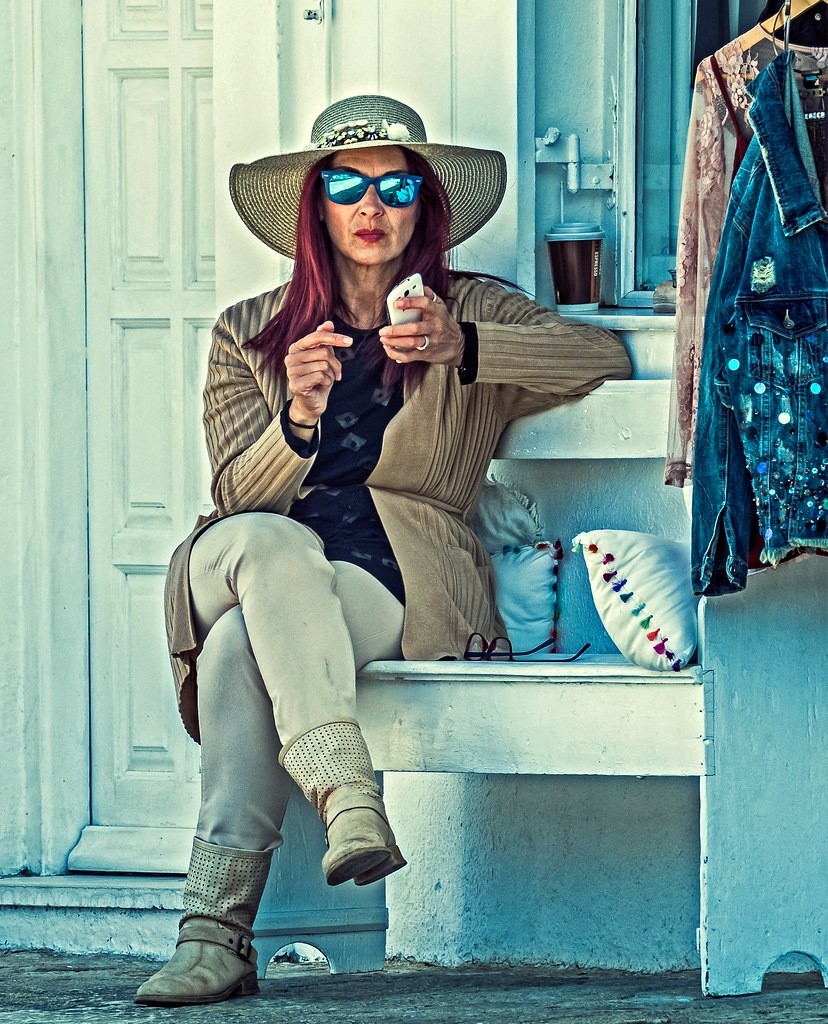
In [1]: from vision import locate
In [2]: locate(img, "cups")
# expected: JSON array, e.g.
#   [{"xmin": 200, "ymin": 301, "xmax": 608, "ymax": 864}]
[{"xmin": 545, "ymin": 220, "xmax": 606, "ymax": 314}]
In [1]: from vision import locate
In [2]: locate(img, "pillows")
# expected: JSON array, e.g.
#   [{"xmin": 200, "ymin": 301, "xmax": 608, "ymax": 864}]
[
  {"xmin": 571, "ymin": 529, "xmax": 698, "ymax": 672},
  {"xmin": 470, "ymin": 473, "xmax": 545, "ymax": 553},
  {"xmin": 490, "ymin": 537, "xmax": 564, "ymax": 655}
]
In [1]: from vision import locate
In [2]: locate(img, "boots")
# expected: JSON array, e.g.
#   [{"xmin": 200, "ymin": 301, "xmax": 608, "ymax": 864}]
[
  {"xmin": 134, "ymin": 836, "xmax": 277, "ymax": 1005},
  {"xmin": 280, "ymin": 722, "xmax": 406, "ymax": 886}
]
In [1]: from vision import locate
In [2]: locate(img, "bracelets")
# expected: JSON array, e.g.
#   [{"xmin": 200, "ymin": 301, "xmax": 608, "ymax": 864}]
[{"xmin": 288, "ymin": 415, "xmax": 320, "ymax": 429}]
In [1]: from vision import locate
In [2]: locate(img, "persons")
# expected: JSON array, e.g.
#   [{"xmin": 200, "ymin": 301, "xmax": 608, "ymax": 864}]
[{"xmin": 135, "ymin": 95, "xmax": 632, "ymax": 1004}]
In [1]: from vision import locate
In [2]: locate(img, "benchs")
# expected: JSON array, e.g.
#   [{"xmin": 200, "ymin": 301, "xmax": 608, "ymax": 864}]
[{"xmin": 356, "ymin": 379, "xmax": 828, "ymax": 999}]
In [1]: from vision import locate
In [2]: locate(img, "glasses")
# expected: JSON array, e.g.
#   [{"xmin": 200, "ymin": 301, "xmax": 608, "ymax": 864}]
[
  {"xmin": 464, "ymin": 632, "xmax": 591, "ymax": 661},
  {"xmin": 320, "ymin": 166, "xmax": 424, "ymax": 209}
]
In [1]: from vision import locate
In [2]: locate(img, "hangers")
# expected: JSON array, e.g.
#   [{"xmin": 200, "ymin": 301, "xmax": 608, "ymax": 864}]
[{"xmin": 696, "ymin": 0, "xmax": 828, "ymax": 83}]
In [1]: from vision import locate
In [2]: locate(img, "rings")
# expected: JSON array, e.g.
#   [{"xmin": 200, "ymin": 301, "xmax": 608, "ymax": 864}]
[
  {"xmin": 416, "ymin": 336, "xmax": 431, "ymax": 351},
  {"xmin": 430, "ymin": 291, "xmax": 438, "ymax": 302}
]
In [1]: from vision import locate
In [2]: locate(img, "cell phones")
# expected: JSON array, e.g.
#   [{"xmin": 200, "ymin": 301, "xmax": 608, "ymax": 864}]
[{"xmin": 385, "ymin": 271, "xmax": 426, "ymax": 364}]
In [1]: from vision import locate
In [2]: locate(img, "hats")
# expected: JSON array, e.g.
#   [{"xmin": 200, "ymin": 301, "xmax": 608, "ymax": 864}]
[{"xmin": 229, "ymin": 95, "xmax": 508, "ymax": 260}]
